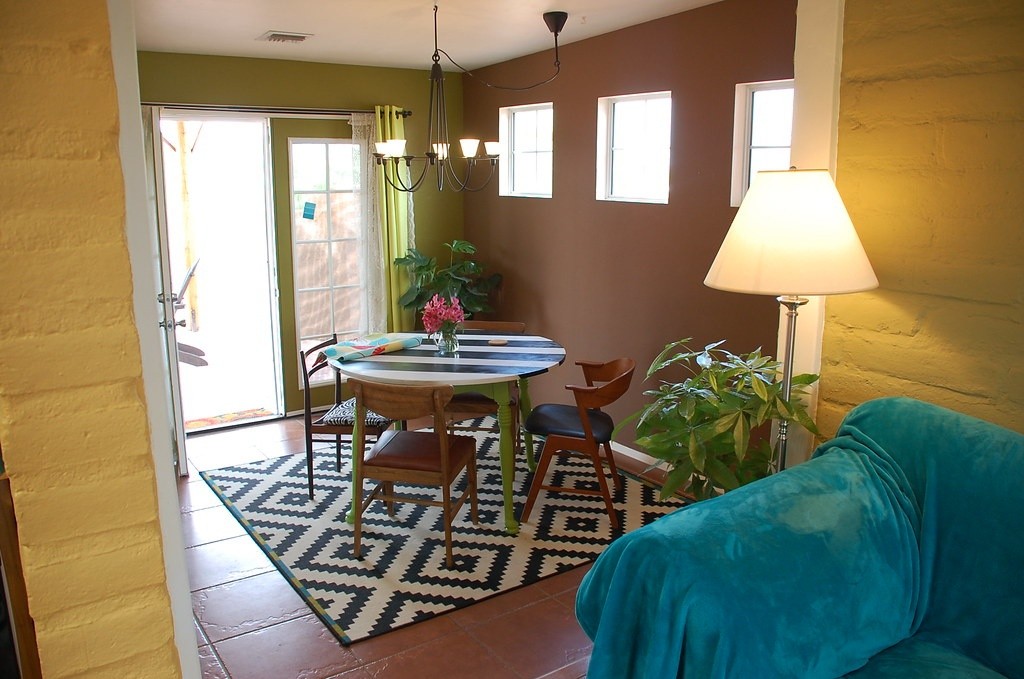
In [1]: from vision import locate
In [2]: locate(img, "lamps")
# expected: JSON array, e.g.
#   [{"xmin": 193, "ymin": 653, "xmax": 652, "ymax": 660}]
[
  {"xmin": 704, "ymin": 167, "xmax": 879, "ymax": 472},
  {"xmin": 372, "ymin": 3, "xmax": 568, "ymax": 193}
]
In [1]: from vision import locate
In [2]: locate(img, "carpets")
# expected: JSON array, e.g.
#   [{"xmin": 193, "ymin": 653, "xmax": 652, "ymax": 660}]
[{"xmin": 198, "ymin": 413, "xmax": 697, "ymax": 647}]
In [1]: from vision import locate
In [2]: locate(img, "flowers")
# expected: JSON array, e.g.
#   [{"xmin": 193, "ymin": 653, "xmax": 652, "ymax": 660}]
[{"xmin": 422, "ymin": 293, "xmax": 465, "ymax": 352}]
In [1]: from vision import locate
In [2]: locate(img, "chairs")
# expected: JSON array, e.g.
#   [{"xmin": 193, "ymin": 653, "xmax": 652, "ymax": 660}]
[
  {"xmin": 520, "ymin": 358, "xmax": 636, "ymax": 528},
  {"xmin": 407, "ymin": 321, "xmax": 526, "ymax": 480},
  {"xmin": 346, "ymin": 378, "xmax": 479, "ymax": 566},
  {"xmin": 300, "ymin": 334, "xmax": 404, "ymax": 506}
]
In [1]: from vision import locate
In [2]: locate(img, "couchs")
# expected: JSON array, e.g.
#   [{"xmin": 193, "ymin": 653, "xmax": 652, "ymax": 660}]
[{"xmin": 577, "ymin": 395, "xmax": 1024, "ymax": 679}]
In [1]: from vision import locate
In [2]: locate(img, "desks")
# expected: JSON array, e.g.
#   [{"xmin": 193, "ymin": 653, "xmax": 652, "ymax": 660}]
[{"xmin": 327, "ymin": 328, "xmax": 567, "ymax": 536}]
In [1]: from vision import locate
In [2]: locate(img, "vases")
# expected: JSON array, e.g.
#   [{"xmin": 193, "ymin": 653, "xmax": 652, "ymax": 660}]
[{"xmin": 433, "ymin": 327, "xmax": 459, "ymax": 353}]
